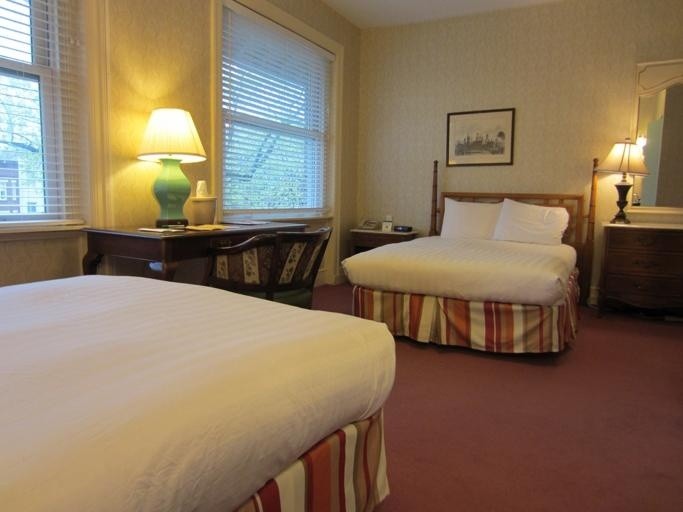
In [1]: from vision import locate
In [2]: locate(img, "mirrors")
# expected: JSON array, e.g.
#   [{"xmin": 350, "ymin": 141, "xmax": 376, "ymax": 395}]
[{"xmin": 626, "ymin": 58, "xmax": 683, "ymax": 225}]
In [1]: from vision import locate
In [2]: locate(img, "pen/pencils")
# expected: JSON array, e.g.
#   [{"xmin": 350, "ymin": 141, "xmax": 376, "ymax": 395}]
[{"xmin": 162, "ymin": 225, "xmax": 185, "ymax": 227}]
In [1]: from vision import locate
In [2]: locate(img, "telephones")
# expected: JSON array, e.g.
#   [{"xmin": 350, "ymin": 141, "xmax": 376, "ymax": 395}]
[{"xmin": 358, "ymin": 218, "xmax": 377, "ymax": 230}]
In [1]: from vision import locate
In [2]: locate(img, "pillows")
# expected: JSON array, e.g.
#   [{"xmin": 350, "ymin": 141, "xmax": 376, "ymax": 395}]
[{"xmin": 440, "ymin": 196, "xmax": 570, "ymax": 247}]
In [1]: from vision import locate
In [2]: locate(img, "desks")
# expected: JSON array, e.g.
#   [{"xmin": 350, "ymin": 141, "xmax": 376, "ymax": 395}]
[{"xmin": 74, "ymin": 219, "xmax": 312, "ymax": 286}]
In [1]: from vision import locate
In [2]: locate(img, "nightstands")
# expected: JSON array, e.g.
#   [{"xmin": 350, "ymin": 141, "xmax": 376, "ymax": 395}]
[
  {"xmin": 589, "ymin": 222, "xmax": 681, "ymax": 321},
  {"xmin": 347, "ymin": 226, "xmax": 419, "ymax": 261}
]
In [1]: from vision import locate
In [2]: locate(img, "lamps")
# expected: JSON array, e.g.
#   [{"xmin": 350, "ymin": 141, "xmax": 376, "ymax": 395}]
[
  {"xmin": 594, "ymin": 141, "xmax": 650, "ymax": 225},
  {"xmin": 134, "ymin": 107, "xmax": 209, "ymax": 227}
]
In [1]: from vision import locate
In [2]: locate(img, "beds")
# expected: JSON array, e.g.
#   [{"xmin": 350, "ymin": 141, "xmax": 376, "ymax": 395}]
[
  {"xmin": 340, "ymin": 155, "xmax": 600, "ymax": 365},
  {"xmin": 1, "ymin": 272, "xmax": 396, "ymax": 511}
]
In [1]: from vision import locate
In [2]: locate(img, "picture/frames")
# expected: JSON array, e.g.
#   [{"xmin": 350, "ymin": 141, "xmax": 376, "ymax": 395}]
[{"xmin": 445, "ymin": 108, "xmax": 516, "ymax": 168}]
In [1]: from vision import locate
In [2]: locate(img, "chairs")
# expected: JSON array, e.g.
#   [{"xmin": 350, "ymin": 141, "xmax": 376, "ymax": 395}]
[{"xmin": 191, "ymin": 224, "xmax": 333, "ymax": 310}]
